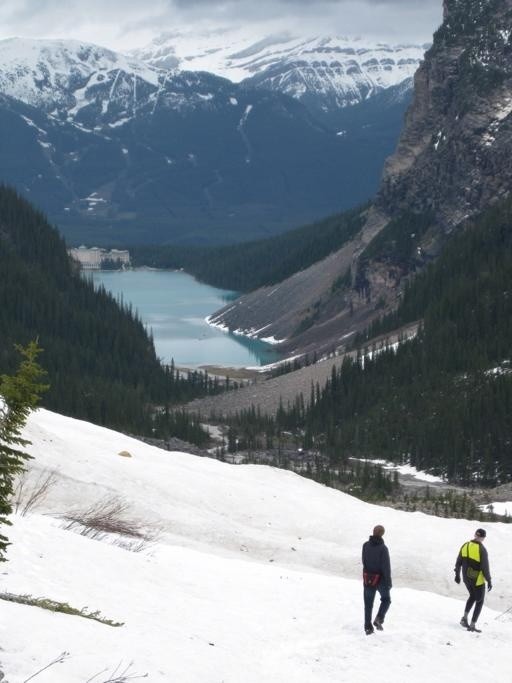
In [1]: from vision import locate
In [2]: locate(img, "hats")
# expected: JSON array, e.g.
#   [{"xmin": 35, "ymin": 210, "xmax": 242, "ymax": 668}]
[{"xmin": 475, "ymin": 529, "xmax": 486, "ymax": 537}]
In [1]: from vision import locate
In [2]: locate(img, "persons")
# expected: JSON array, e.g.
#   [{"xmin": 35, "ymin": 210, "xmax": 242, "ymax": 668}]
[
  {"xmin": 454, "ymin": 529, "xmax": 493, "ymax": 633},
  {"xmin": 361, "ymin": 526, "xmax": 393, "ymax": 636}
]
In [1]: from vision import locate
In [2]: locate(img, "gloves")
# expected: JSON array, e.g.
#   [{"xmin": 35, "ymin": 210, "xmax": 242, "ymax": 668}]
[
  {"xmin": 455, "ymin": 575, "xmax": 460, "ymax": 583},
  {"xmin": 488, "ymin": 580, "xmax": 492, "ymax": 591}
]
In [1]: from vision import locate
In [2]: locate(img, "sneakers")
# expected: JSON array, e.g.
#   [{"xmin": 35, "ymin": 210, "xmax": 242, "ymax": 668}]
[
  {"xmin": 460, "ymin": 617, "xmax": 481, "ymax": 632},
  {"xmin": 366, "ymin": 618, "xmax": 383, "ymax": 635}
]
